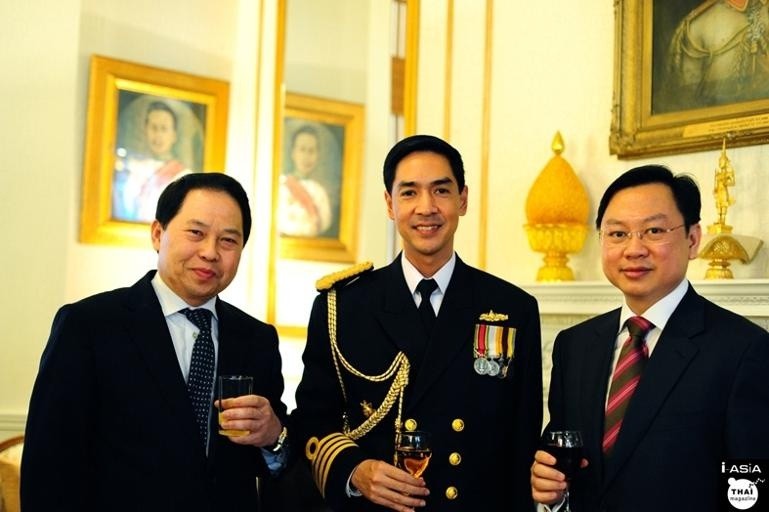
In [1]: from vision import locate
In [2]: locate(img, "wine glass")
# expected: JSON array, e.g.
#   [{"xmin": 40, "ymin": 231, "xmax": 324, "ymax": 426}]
[
  {"xmin": 396, "ymin": 429, "xmax": 432, "ymax": 510},
  {"xmin": 544, "ymin": 431, "xmax": 585, "ymax": 512}
]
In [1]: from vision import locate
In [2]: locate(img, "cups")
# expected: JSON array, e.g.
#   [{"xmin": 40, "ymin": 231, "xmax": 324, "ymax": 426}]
[{"xmin": 218, "ymin": 375, "xmax": 253, "ymax": 436}]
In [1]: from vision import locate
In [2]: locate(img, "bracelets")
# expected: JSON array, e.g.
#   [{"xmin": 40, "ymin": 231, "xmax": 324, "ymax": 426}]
[{"xmin": 272, "ymin": 421, "xmax": 287, "ymax": 456}]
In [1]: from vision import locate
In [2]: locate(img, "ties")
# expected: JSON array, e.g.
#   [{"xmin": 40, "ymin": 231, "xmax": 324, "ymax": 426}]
[
  {"xmin": 602, "ymin": 316, "xmax": 656, "ymax": 456},
  {"xmin": 178, "ymin": 307, "xmax": 215, "ymax": 454},
  {"xmin": 416, "ymin": 279, "xmax": 438, "ymax": 340}
]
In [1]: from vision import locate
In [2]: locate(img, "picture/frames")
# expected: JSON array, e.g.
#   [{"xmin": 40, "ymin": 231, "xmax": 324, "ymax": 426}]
[
  {"xmin": 267, "ymin": 92, "xmax": 367, "ymax": 264},
  {"xmin": 607, "ymin": 0, "xmax": 769, "ymax": 160},
  {"xmin": 77, "ymin": 52, "xmax": 231, "ymax": 251}
]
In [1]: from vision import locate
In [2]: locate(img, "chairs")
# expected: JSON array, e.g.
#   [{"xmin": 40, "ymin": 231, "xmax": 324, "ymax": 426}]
[{"xmin": 1, "ymin": 435, "xmax": 26, "ymax": 512}]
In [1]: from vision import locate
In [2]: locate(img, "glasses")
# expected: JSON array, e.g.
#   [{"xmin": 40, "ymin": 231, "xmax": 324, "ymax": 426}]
[{"xmin": 598, "ymin": 224, "xmax": 686, "ymax": 250}]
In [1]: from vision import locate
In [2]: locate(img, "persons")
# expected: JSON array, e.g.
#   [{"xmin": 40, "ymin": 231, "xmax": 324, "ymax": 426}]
[
  {"xmin": 652, "ymin": 0, "xmax": 769, "ymax": 113},
  {"xmin": 279, "ymin": 123, "xmax": 332, "ymax": 238},
  {"xmin": 17, "ymin": 170, "xmax": 290, "ymax": 509},
  {"xmin": 529, "ymin": 160, "xmax": 769, "ymax": 510},
  {"xmin": 289, "ymin": 133, "xmax": 546, "ymax": 510},
  {"xmin": 117, "ymin": 101, "xmax": 194, "ymax": 223}
]
